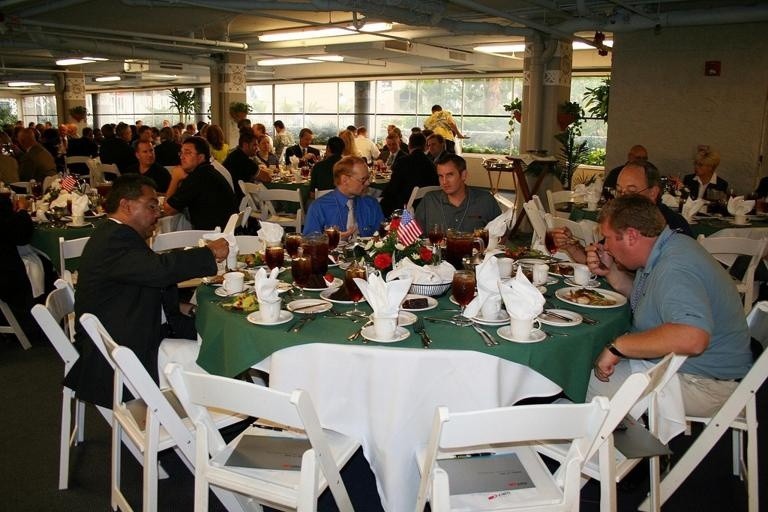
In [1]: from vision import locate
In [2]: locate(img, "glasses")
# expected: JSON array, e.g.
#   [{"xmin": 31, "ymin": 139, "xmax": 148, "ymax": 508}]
[
  {"xmin": 140, "ymin": 198, "xmax": 161, "ymax": 211},
  {"xmin": 345, "ymin": 173, "xmax": 371, "ymax": 183},
  {"xmin": 613, "ymin": 184, "xmax": 653, "ymax": 199}
]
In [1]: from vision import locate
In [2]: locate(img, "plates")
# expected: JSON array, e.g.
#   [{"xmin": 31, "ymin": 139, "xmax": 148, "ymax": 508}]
[
  {"xmin": 582, "ymin": 207, "xmax": 601, "ymax": 212},
  {"xmin": 200, "ymin": 236, "xmax": 627, "ymax": 344},
  {"xmin": 66, "ymin": 221, "xmax": 92, "ymax": 227},
  {"xmin": 730, "ymin": 222, "xmax": 752, "ymax": 227}
]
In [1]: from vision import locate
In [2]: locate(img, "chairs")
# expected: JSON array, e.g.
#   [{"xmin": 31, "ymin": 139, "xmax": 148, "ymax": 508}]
[
  {"xmin": 79, "ymin": 312, "xmax": 249, "ymax": 512},
  {"xmin": 415, "ymin": 394, "xmax": 609, "ymax": 512},
  {"xmin": 530, "ymin": 351, "xmax": 688, "ymax": 512},
  {"xmin": 163, "ymin": 363, "xmax": 361, "ymax": 512},
  {"xmin": 638, "ymin": 301, "xmax": 768, "ymax": 512}
]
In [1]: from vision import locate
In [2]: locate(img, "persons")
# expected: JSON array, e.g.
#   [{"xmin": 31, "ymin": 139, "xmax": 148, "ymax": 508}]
[{"xmin": 2, "ymin": 105, "xmax": 768, "ymax": 504}]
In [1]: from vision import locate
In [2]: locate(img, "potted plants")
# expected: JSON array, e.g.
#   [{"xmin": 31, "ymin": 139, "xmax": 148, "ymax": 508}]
[
  {"xmin": 229, "ymin": 101, "xmax": 253, "ymax": 122},
  {"xmin": 69, "ymin": 106, "xmax": 87, "ymax": 122}
]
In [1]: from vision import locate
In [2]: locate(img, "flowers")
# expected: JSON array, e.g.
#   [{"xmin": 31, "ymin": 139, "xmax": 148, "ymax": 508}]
[
  {"xmin": 553, "ymin": 101, "xmax": 590, "ymax": 188},
  {"xmin": 504, "ymin": 97, "xmax": 522, "ymax": 140}
]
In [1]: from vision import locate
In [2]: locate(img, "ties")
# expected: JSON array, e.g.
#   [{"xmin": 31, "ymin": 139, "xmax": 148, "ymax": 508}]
[
  {"xmin": 346, "ymin": 198, "xmax": 360, "ymax": 243},
  {"xmin": 387, "ymin": 153, "xmax": 394, "ymax": 166},
  {"xmin": 302, "ymin": 148, "xmax": 306, "ymax": 155}
]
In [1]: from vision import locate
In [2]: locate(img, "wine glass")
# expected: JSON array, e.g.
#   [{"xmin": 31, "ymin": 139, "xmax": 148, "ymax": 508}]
[
  {"xmin": 67, "ymin": 195, "xmax": 73, "ymax": 214},
  {"xmin": 265, "ymin": 224, "xmax": 490, "ymax": 326},
  {"xmin": 301, "ymin": 166, "xmax": 309, "ymax": 179}
]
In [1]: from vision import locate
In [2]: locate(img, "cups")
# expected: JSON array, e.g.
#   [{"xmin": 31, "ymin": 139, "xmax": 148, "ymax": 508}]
[
  {"xmin": 588, "ymin": 202, "xmax": 598, "ymax": 209},
  {"xmin": 72, "ymin": 214, "xmax": 84, "ymax": 225},
  {"xmin": 735, "ymin": 215, "xmax": 745, "ymax": 224},
  {"xmin": 699, "ymin": 206, "xmax": 707, "ymax": 213}
]
